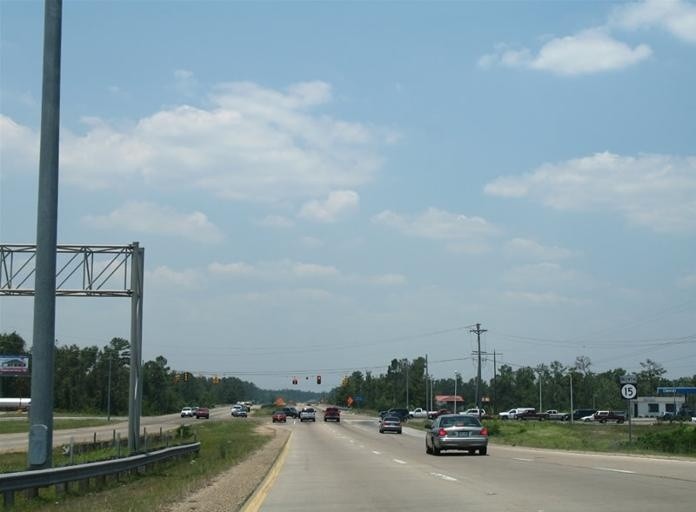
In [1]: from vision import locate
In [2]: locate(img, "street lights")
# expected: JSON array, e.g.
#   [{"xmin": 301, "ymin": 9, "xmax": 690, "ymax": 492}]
[
  {"xmin": 453, "ymin": 369, "xmax": 458, "ymax": 414},
  {"xmin": 538, "ymin": 371, "xmax": 542, "ymax": 413}
]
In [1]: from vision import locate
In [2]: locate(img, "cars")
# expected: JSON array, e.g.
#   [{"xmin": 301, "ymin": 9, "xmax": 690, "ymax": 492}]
[
  {"xmin": 676, "ymin": 412, "xmax": 691, "ymax": 422},
  {"xmin": 427, "ymin": 409, "xmax": 450, "ymax": 419},
  {"xmin": 191, "ymin": 407, "xmax": 199, "ymax": 414},
  {"xmin": 389, "ymin": 428, "xmax": 393, "ymax": 429},
  {"xmin": 424, "ymin": 413, "xmax": 488, "ymax": 456},
  {"xmin": 180, "ymin": 407, "xmax": 193, "ymax": 417},
  {"xmin": 378, "ymin": 417, "xmax": 401, "ymax": 434},
  {"xmin": 229, "ymin": 401, "xmax": 251, "ymax": 417},
  {"xmin": 272, "ymin": 411, "xmax": 286, "ymax": 422}
]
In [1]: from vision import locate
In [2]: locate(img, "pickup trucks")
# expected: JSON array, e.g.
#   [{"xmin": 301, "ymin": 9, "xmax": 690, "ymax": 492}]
[
  {"xmin": 497, "ymin": 408, "xmax": 626, "ymax": 425},
  {"xmin": 408, "ymin": 408, "xmax": 437, "ymax": 419}
]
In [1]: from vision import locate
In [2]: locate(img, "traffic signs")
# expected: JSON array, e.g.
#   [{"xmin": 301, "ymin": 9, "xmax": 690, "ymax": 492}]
[
  {"xmin": 618, "ymin": 376, "xmax": 638, "ymax": 386},
  {"xmin": 619, "ymin": 384, "xmax": 637, "ymax": 400}
]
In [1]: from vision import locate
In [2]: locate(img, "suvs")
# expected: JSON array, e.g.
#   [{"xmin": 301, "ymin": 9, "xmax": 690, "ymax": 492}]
[
  {"xmin": 380, "ymin": 408, "xmax": 408, "ymax": 423},
  {"xmin": 278, "ymin": 407, "xmax": 298, "ymax": 418},
  {"xmin": 195, "ymin": 408, "xmax": 208, "ymax": 419},
  {"xmin": 324, "ymin": 408, "xmax": 340, "ymax": 422},
  {"xmin": 459, "ymin": 409, "xmax": 485, "ymax": 419},
  {"xmin": 299, "ymin": 407, "xmax": 316, "ymax": 422},
  {"xmin": 656, "ymin": 412, "xmax": 673, "ymax": 421}
]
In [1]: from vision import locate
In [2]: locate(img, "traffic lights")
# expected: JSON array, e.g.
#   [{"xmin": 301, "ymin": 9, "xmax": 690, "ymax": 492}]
[{"xmin": 316, "ymin": 376, "xmax": 320, "ymax": 384}]
